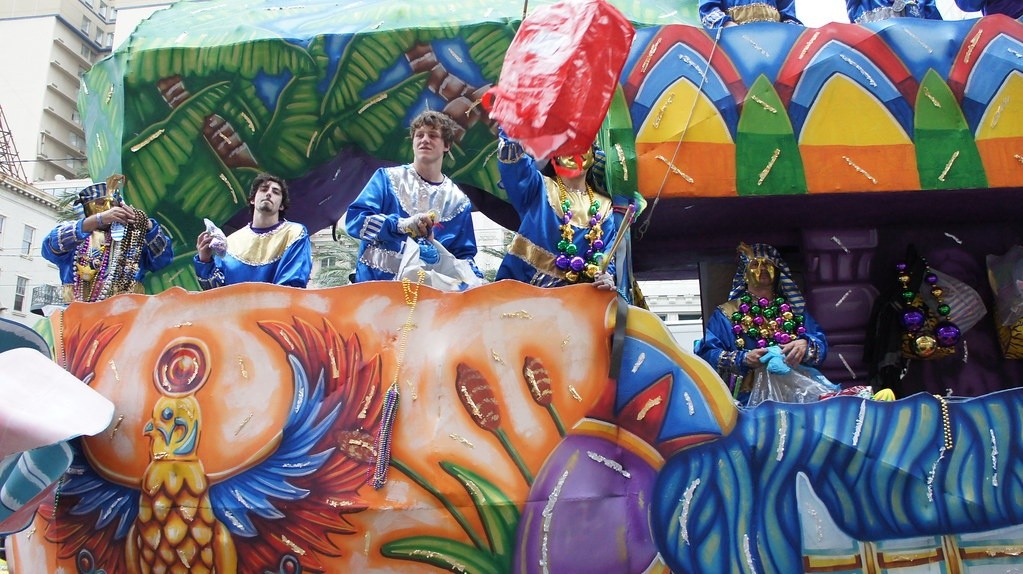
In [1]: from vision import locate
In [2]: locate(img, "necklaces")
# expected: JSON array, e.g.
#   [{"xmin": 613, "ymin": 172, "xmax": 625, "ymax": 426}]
[
  {"xmin": 735, "ymin": 291, "xmax": 803, "ymax": 352},
  {"xmin": 556, "ymin": 178, "xmax": 605, "ymax": 281},
  {"xmin": 395, "ymin": 272, "xmax": 426, "ymax": 382},
  {"xmin": 368, "ymin": 384, "xmax": 402, "ymax": 490},
  {"xmin": 71, "ymin": 208, "xmax": 148, "ymax": 303},
  {"xmin": 896, "ymin": 256, "xmax": 960, "ymax": 357}
]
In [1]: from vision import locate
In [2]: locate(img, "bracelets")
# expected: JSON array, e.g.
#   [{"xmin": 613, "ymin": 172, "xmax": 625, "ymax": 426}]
[{"xmin": 97, "ymin": 213, "xmax": 103, "ymax": 227}]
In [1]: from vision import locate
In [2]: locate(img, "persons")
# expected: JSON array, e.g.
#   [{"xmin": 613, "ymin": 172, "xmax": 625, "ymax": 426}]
[
  {"xmin": 700, "ymin": 0, "xmax": 1023, "ymax": 29},
  {"xmin": 346, "ymin": 111, "xmax": 481, "ymax": 294},
  {"xmin": 195, "ymin": 173, "xmax": 312, "ymax": 291},
  {"xmin": 491, "ymin": 123, "xmax": 616, "ymax": 291},
  {"xmin": 41, "ymin": 183, "xmax": 174, "ymax": 308},
  {"xmin": 694, "ymin": 244, "xmax": 828, "ymax": 408}
]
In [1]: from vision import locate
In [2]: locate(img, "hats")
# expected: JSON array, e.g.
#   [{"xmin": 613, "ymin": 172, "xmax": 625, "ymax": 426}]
[{"xmin": 57, "ymin": 182, "xmax": 123, "ymax": 222}]
